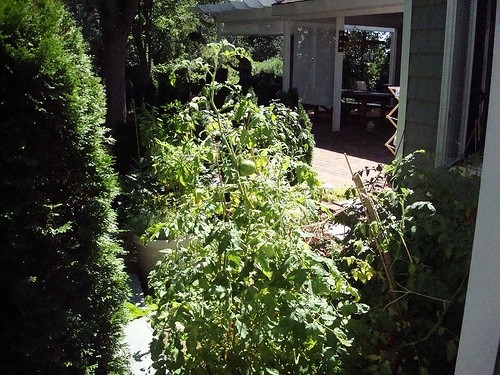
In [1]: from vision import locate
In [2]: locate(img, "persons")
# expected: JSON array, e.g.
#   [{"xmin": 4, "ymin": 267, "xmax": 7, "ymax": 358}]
[{"xmin": 226, "ymin": 50, "xmax": 254, "ymax": 99}]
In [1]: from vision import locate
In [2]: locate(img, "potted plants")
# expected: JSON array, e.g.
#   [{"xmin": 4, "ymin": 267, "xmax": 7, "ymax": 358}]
[{"xmin": 124, "ymin": 157, "xmax": 195, "ymax": 297}]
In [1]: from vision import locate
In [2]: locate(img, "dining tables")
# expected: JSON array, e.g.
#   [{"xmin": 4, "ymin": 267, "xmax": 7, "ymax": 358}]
[{"xmin": 340, "ymin": 91, "xmax": 393, "ymax": 127}]
